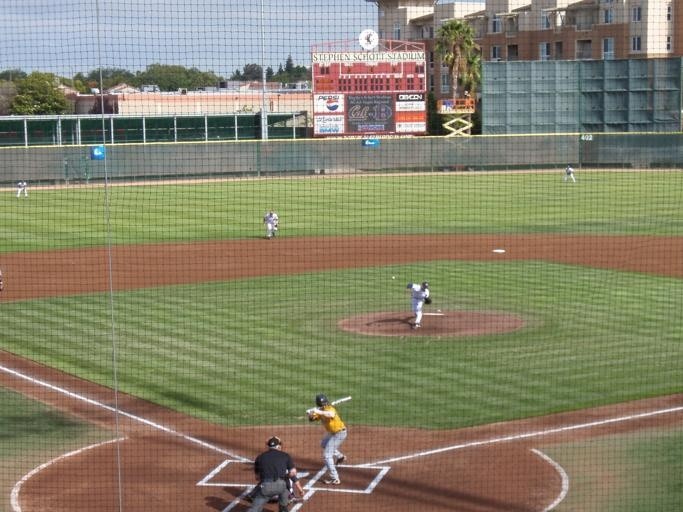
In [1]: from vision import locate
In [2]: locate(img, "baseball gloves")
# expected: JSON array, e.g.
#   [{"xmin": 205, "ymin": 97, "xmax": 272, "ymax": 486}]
[{"xmin": 424, "ymin": 297, "xmax": 432, "ymax": 304}]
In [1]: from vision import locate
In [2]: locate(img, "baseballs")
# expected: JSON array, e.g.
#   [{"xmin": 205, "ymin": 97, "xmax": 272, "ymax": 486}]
[{"xmin": 392, "ymin": 276, "xmax": 396, "ymax": 280}]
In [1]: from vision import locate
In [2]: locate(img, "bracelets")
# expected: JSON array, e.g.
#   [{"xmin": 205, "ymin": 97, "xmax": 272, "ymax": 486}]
[{"xmin": 299, "ymin": 491, "xmax": 303, "ymax": 493}]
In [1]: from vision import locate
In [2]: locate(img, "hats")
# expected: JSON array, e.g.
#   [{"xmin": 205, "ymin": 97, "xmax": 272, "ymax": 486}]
[{"xmin": 268, "ymin": 437, "xmax": 282, "ymax": 447}]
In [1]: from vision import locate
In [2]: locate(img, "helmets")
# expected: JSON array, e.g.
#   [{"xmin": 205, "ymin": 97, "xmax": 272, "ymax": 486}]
[
  {"xmin": 422, "ymin": 282, "xmax": 428, "ymax": 289},
  {"xmin": 316, "ymin": 394, "xmax": 328, "ymax": 407}
]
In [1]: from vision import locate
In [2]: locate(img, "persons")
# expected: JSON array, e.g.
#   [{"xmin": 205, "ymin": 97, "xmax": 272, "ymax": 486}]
[
  {"xmin": 562, "ymin": 164, "xmax": 575, "ymax": 183},
  {"xmin": 404, "ymin": 280, "xmax": 429, "ymax": 330},
  {"xmin": 15, "ymin": 179, "xmax": 29, "ymax": 198},
  {"xmin": 244, "ymin": 468, "xmax": 305, "ymax": 505},
  {"xmin": 244, "ymin": 434, "xmax": 298, "ymax": 512},
  {"xmin": 262, "ymin": 210, "xmax": 279, "ymax": 239},
  {"xmin": 306, "ymin": 393, "xmax": 348, "ymax": 486}
]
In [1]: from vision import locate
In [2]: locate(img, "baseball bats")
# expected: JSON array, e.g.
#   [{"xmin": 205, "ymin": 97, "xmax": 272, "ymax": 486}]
[{"xmin": 304, "ymin": 395, "xmax": 353, "ymax": 415}]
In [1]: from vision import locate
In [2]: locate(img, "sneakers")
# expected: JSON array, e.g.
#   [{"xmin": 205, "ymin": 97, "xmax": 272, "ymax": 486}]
[
  {"xmin": 336, "ymin": 457, "xmax": 346, "ymax": 465},
  {"xmin": 323, "ymin": 478, "xmax": 340, "ymax": 485},
  {"xmin": 412, "ymin": 323, "xmax": 421, "ymax": 330}
]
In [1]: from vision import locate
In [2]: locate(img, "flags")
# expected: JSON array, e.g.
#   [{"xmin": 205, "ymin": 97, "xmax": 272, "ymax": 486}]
[{"xmin": 88, "ymin": 145, "xmax": 105, "ymax": 161}]
